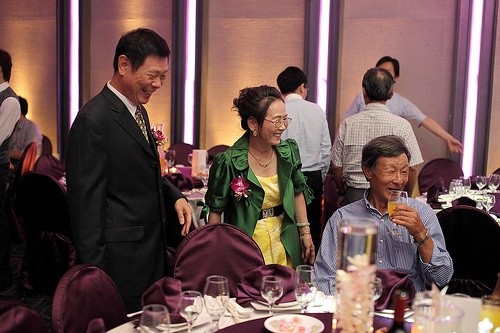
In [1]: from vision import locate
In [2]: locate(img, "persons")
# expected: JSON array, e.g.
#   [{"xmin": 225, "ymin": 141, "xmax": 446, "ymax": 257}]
[
  {"xmin": 9, "ymin": 97, "xmax": 43, "ymax": 180},
  {"xmin": 328, "ymin": 68, "xmax": 425, "ymax": 207},
  {"xmin": 278, "ymin": 66, "xmax": 333, "ymax": 254},
  {"xmin": 205, "ymin": 85, "xmax": 315, "ymax": 278},
  {"xmin": 310, "ymin": 135, "xmax": 453, "ymax": 297},
  {"xmin": 344, "ymin": 56, "xmax": 464, "ymax": 154},
  {"xmin": 0, "ymin": 49, "xmax": 21, "ymax": 198},
  {"xmin": 65, "ymin": 29, "xmax": 194, "ymax": 317}
]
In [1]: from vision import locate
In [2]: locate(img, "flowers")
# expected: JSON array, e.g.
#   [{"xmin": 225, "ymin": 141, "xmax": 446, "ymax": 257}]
[
  {"xmin": 230, "ymin": 176, "xmax": 251, "ymax": 201},
  {"xmin": 151, "ymin": 128, "xmax": 168, "ymax": 146}
]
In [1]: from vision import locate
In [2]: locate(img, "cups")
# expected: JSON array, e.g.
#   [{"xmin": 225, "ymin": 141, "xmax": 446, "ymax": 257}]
[
  {"xmin": 411, "ymin": 301, "xmax": 465, "ymax": 333},
  {"xmin": 373, "ymin": 277, "xmax": 382, "ymax": 301},
  {"xmin": 479, "ymin": 295, "xmax": 500, "ymax": 333},
  {"xmin": 452, "ymin": 293, "xmax": 471, "ymax": 299},
  {"xmin": 388, "ymin": 190, "xmax": 408, "ymax": 220},
  {"xmin": 139, "ymin": 304, "xmax": 171, "ymax": 333}
]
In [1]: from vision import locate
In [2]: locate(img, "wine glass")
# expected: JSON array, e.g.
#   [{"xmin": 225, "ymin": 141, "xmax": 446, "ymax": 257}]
[
  {"xmin": 204, "ymin": 275, "xmax": 229, "ymax": 333},
  {"xmin": 449, "ymin": 173, "xmax": 500, "ymax": 214},
  {"xmin": 200, "ymin": 164, "xmax": 209, "ymax": 193},
  {"xmin": 261, "ymin": 276, "xmax": 283, "ymax": 316},
  {"xmin": 177, "ymin": 291, "xmax": 203, "ymax": 333},
  {"xmin": 294, "ymin": 265, "xmax": 318, "ymax": 315},
  {"xmin": 164, "ymin": 150, "xmax": 175, "ymax": 173}
]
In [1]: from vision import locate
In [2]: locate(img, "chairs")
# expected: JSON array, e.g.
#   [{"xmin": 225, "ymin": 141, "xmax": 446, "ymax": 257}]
[{"xmin": 0, "ymin": 134, "xmax": 500, "ymax": 333}]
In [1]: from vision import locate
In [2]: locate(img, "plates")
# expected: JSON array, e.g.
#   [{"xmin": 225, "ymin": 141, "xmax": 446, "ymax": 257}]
[
  {"xmin": 264, "ymin": 314, "xmax": 325, "ymax": 333},
  {"xmin": 249, "ymin": 290, "xmax": 324, "ymax": 311},
  {"xmin": 157, "ymin": 314, "xmax": 198, "ymax": 327}
]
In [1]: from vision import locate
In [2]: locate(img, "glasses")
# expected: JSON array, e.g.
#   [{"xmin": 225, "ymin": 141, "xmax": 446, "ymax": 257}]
[{"xmin": 265, "ymin": 116, "xmax": 292, "ymax": 128}]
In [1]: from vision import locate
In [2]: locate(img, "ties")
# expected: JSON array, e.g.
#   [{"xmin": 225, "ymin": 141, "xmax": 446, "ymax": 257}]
[{"xmin": 135, "ymin": 106, "xmax": 148, "ymax": 142}]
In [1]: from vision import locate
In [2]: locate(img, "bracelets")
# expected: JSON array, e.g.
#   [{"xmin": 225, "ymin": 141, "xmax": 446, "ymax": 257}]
[
  {"xmin": 414, "ymin": 230, "xmax": 430, "ymax": 246},
  {"xmin": 296, "ymin": 223, "xmax": 310, "ymax": 226},
  {"xmin": 300, "ymin": 232, "xmax": 310, "ymax": 236}
]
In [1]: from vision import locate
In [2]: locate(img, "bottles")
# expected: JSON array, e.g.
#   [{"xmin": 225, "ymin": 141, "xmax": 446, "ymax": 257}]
[{"xmin": 387, "ymin": 290, "xmax": 410, "ymax": 333}]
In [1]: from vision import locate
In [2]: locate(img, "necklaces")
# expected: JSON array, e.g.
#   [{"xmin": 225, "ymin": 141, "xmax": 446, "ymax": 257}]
[{"xmin": 247, "ymin": 149, "xmax": 274, "ymax": 167}]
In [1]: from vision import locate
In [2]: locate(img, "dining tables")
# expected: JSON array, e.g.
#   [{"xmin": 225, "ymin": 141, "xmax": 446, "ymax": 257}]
[
  {"xmin": 416, "ymin": 189, "xmax": 500, "ymax": 227},
  {"xmin": 100, "ymin": 288, "xmax": 414, "ymax": 333},
  {"xmin": 167, "ymin": 166, "xmax": 224, "ymax": 232}
]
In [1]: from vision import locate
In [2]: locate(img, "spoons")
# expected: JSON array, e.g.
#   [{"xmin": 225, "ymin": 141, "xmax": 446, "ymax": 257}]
[{"xmin": 229, "ymin": 299, "xmax": 254, "ymax": 315}]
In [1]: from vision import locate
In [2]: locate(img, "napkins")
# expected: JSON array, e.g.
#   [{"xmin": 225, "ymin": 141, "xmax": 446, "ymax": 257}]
[
  {"xmin": 451, "ymin": 196, "xmax": 476, "ymax": 208},
  {"xmin": 373, "ymin": 269, "xmax": 416, "ymax": 312},
  {"xmin": 235, "ymin": 264, "xmax": 311, "ymax": 308},
  {"xmin": 427, "ymin": 184, "xmax": 447, "ymax": 209},
  {"xmin": 141, "ymin": 277, "xmax": 194, "ymax": 326},
  {"xmin": 436, "ymin": 178, "xmax": 448, "ymax": 196}
]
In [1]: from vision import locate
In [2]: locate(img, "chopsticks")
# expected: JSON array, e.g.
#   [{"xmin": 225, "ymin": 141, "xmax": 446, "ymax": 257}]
[{"xmin": 221, "ymin": 296, "xmax": 242, "ymax": 324}]
